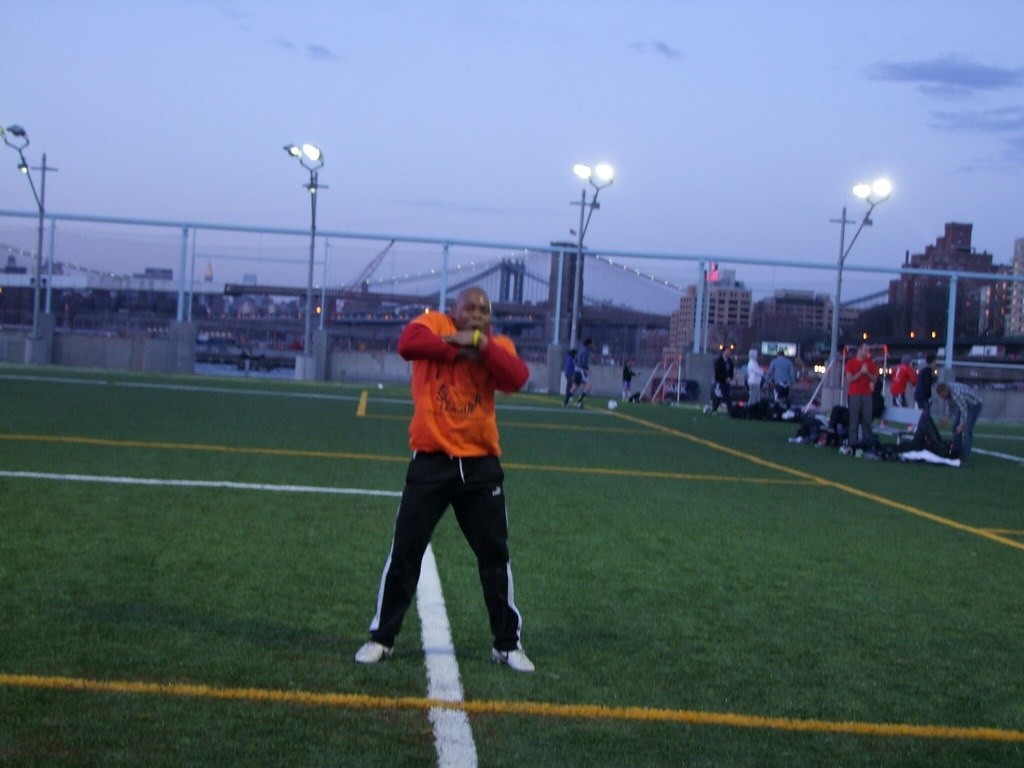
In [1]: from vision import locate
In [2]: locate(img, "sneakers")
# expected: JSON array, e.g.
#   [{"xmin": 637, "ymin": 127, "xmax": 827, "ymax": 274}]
[
  {"xmin": 354, "ymin": 641, "xmax": 395, "ymax": 664},
  {"xmin": 492, "ymin": 647, "xmax": 536, "ymax": 671}
]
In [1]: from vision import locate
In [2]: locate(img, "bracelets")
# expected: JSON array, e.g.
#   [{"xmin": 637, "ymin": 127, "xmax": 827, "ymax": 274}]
[{"xmin": 473, "ymin": 329, "xmax": 479, "ymax": 346}]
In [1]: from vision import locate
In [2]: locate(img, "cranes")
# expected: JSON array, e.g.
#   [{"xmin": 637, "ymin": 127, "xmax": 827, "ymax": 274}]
[{"xmin": 337, "ymin": 239, "xmax": 395, "ymax": 310}]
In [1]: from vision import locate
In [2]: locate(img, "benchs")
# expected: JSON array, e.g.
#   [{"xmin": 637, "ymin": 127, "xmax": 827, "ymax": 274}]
[{"xmin": 869, "ymin": 407, "xmax": 925, "ymax": 446}]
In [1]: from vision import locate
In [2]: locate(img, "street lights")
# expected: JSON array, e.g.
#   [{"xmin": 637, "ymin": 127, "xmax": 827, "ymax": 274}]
[
  {"xmin": 821, "ymin": 178, "xmax": 892, "ymax": 411},
  {"xmin": 283, "ymin": 144, "xmax": 329, "ymax": 381},
  {"xmin": 0, "ymin": 123, "xmax": 57, "ymax": 364},
  {"xmin": 560, "ymin": 164, "xmax": 613, "ymax": 395}
]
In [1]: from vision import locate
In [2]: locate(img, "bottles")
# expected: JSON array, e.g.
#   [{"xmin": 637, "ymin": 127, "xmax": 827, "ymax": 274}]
[{"xmin": 790, "ymin": 438, "xmax": 805, "ymax": 443}]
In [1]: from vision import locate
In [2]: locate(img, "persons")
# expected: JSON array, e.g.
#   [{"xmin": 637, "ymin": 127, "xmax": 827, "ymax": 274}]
[
  {"xmin": 936, "ymin": 382, "xmax": 982, "ymax": 457},
  {"xmin": 712, "ymin": 347, "xmax": 735, "ymax": 411},
  {"xmin": 766, "ymin": 350, "xmax": 794, "ymax": 401},
  {"xmin": 621, "ymin": 359, "xmax": 636, "ymax": 400},
  {"xmin": 563, "ymin": 350, "xmax": 577, "ymax": 404},
  {"xmin": 354, "ymin": 288, "xmax": 536, "ymax": 672},
  {"xmin": 569, "ymin": 338, "xmax": 592, "ymax": 408},
  {"xmin": 891, "ymin": 355, "xmax": 916, "ymax": 407},
  {"xmin": 748, "ymin": 349, "xmax": 764, "ymax": 406},
  {"xmin": 913, "ymin": 354, "xmax": 941, "ymax": 413},
  {"xmin": 829, "ymin": 378, "xmax": 884, "ymax": 444},
  {"xmin": 844, "ymin": 341, "xmax": 878, "ymax": 447}
]
